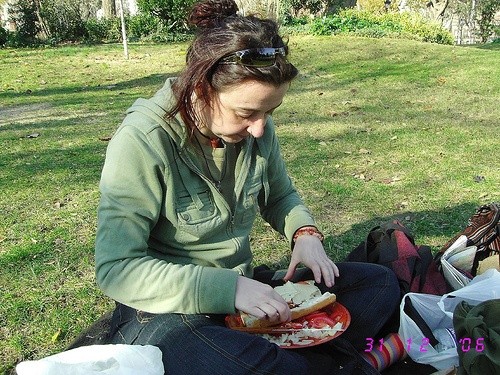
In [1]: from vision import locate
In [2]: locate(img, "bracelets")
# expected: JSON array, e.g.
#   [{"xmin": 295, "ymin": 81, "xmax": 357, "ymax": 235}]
[{"xmin": 293, "ymin": 227, "xmax": 323, "ymax": 243}]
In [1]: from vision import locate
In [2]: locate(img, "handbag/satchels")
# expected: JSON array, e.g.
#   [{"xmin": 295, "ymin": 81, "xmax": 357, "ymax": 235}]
[
  {"xmin": 399, "ymin": 268, "xmax": 500, "ymax": 369},
  {"xmin": 421, "ymin": 200, "xmax": 500, "ymax": 296},
  {"xmin": 452, "ymin": 297, "xmax": 500, "ymax": 374}
]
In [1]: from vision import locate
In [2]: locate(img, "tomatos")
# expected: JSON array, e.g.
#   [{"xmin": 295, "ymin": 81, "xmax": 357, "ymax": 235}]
[{"xmin": 305, "ymin": 311, "xmax": 335, "ymax": 328}]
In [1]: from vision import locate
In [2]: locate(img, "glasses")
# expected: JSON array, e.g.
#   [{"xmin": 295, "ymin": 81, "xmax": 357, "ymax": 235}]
[{"xmin": 218, "ymin": 46, "xmax": 292, "ymax": 68}]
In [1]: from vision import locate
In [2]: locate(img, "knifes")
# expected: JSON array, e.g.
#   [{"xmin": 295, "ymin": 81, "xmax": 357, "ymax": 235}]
[{"xmin": 232, "ymin": 326, "xmax": 347, "ymax": 332}]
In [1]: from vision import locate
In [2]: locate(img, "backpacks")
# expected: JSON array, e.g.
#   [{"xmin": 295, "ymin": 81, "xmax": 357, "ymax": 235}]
[{"xmin": 343, "ymin": 223, "xmax": 449, "ymax": 298}]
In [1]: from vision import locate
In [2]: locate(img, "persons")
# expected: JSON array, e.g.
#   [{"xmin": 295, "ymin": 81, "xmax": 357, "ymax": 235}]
[{"xmin": 95, "ymin": 0, "xmax": 409, "ymax": 375}]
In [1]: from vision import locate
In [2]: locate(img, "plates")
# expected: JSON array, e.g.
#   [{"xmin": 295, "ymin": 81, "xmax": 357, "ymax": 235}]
[{"xmin": 225, "ymin": 300, "xmax": 351, "ymax": 348}]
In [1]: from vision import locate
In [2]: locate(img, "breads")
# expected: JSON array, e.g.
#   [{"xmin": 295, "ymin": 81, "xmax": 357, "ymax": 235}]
[{"xmin": 240, "ymin": 279, "xmax": 337, "ymax": 328}]
